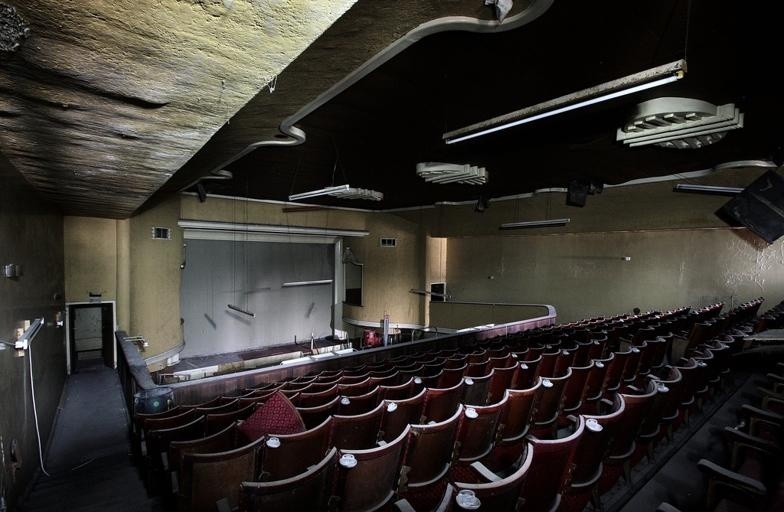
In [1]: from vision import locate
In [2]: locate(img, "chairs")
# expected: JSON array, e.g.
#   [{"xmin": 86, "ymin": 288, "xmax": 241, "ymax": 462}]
[{"xmin": 137, "ymin": 298, "xmax": 783, "ymax": 511}]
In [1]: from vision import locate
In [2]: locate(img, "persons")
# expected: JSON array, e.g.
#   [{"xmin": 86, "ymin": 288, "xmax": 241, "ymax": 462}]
[{"xmin": 632, "ymin": 307, "xmax": 642, "ymax": 315}]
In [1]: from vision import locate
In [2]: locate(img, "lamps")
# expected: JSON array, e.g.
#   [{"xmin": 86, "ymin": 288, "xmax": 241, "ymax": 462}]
[
  {"xmin": 653, "ymin": 149, "xmax": 745, "ymax": 194},
  {"xmin": 228, "ymin": 190, "xmax": 255, "ymax": 317},
  {"xmin": 498, "ymin": 163, "xmax": 571, "ymax": 229},
  {"xmin": 409, "ymin": 149, "xmax": 451, "ymax": 299},
  {"xmin": 271, "ymin": 154, "xmax": 335, "ymax": 288},
  {"xmin": 180, "ymin": 244, "xmax": 188, "ymax": 271}
]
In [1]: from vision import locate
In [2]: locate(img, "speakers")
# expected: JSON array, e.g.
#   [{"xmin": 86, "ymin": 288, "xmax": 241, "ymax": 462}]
[
  {"xmin": 724, "ymin": 171, "xmax": 784, "ymax": 244},
  {"xmin": 565, "ymin": 178, "xmax": 589, "ymax": 208}
]
